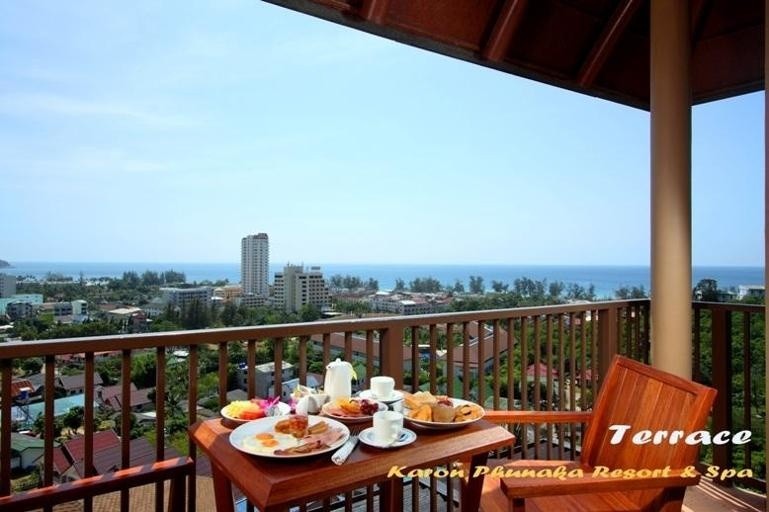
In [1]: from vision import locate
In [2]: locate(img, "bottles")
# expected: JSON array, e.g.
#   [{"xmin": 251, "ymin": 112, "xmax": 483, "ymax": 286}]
[{"xmin": 323, "ymin": 358, "xmax": 353, "ymax": 400}]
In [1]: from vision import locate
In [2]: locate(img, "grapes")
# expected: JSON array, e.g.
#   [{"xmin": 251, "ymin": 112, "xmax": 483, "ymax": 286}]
[{"xmin": 360, "ymin": 400, "xmax": 379, "ymax": 415}]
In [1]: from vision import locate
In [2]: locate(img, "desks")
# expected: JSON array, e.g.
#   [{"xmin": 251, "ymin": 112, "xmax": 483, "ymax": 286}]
[{"xmin": 188, "ymin": 390, "xmax": 516, "ymax": 512}]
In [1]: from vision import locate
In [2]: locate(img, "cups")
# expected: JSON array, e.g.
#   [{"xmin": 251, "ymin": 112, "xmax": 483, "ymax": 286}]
[{"xmin": 370, "ymin": 375, "xmax": 397, "ymax": 398}]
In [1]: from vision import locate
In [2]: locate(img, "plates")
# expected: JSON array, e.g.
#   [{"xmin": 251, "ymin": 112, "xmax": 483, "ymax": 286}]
[{"xmin": 220, "ymin": 390, "xmax": 485, "ymax": 459}]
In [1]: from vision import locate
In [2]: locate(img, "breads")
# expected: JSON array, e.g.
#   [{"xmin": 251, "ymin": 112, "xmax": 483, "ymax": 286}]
[{"xmin": 404, "ymin": 389, "xmax": 483, "ymax": 423}]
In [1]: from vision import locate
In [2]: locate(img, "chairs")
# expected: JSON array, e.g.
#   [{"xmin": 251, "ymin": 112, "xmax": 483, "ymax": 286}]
[
  {"xmin": 466, "ymin": 354, "xmax": 717, "ymax": 512},
  {"xmin": 0, "ymin": 456, "xmax": 195, "ymax": 512}
]
